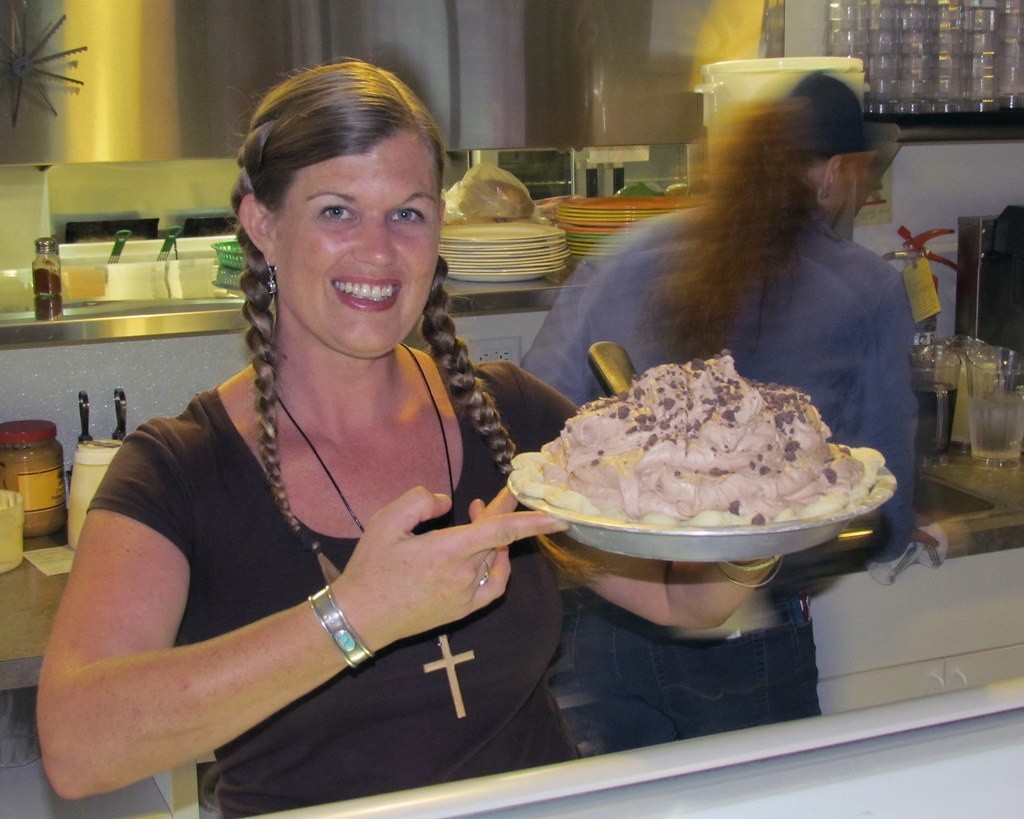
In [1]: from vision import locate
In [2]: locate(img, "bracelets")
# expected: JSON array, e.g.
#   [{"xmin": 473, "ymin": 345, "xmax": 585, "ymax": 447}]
[
  {"xmin": 716, "ymin": 559, "xmax": 782, "ymax": 588},
  {"xmin": 308, "ymin": 585, "xmax": 375, "ymax": 669},
  {"xmin": 726, "ymin": 556, "xmax": 779, "ymax": 572}
]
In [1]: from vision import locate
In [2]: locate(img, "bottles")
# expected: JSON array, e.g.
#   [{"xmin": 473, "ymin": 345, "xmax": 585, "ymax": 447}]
[
  {"xmin": 0, "ymin": 419, "xmax": 69, "ymax": 538},
  {"xmin": 32, "ymin": 237, "xmax": 64, "ymax": 323}
]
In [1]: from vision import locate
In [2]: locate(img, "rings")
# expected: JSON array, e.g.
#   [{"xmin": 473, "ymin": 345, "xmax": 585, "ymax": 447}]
[{"xmin": 480, "ymin": 561, "xmax": 490, "ymax": 586}]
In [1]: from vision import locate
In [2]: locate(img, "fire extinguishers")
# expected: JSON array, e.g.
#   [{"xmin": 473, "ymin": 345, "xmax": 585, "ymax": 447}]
[{"xmin": 882, "ymin": 224, "xmax": 959, "ymax": 295}]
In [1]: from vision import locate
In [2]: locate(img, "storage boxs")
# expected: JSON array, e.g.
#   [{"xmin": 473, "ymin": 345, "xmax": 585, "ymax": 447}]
[{"xmin": 59, "ymin": 234, "xmax": 240, "ymax": 298}]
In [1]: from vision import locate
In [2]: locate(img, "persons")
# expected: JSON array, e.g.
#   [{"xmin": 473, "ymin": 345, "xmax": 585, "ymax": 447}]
[
  {"xmin": 34, "ymin": 58, "xmax": 785, "ymax": 819},
  {"xmin": 520, "ymin": 68, "xmax": 940, "ymax": 761}
]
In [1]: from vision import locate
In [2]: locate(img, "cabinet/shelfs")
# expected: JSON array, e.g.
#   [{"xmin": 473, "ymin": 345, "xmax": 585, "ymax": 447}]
[{"xmin": 806, "ymin": 524, "xmax": 1023, "ymax": 714}]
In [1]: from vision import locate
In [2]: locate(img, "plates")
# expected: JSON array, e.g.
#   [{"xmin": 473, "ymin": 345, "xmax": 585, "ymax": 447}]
[
  {"xmin": 437, "ymin": 197, "xmax": 687, "ymax": 282},
  {"xmin": 507, "ymin": 459, "xmax": 897, "ymax": 562}
]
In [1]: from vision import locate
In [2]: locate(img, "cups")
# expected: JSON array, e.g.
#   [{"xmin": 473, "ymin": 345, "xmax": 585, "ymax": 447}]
[
  {"xmin": 0, "ymin": 490, "xmax": 24, "ymax": 572},
  {"xmin": 908, "ymin": 336, "xmax": 1024, "ymax": 467}
]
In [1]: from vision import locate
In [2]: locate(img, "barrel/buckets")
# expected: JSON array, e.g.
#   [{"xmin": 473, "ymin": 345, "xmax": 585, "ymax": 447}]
[{"xmin": 695, "ymin": 55, "xmax": 870, "ymax": 168}]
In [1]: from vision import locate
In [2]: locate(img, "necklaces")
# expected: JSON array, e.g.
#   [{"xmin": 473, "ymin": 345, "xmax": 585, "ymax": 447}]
[{"xmin": 276, "ymin": 342, "xmax": 455, "ymax": 535}]
